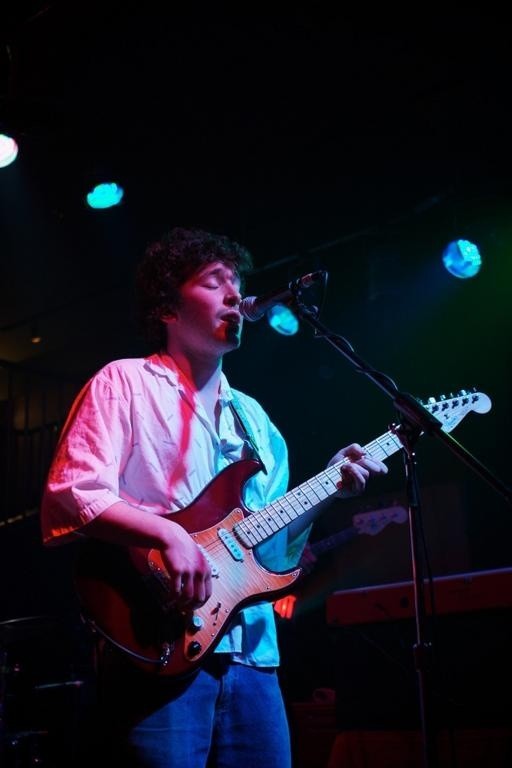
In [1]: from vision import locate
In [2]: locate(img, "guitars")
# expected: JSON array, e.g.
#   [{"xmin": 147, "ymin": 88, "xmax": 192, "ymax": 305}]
[
  {"xmin": 76, "ymin": 389, "xmax": 493, "ymax": 674},
  {"xmin": 301, "ymin": 502, "xmax": 408, "ymax": 573}
]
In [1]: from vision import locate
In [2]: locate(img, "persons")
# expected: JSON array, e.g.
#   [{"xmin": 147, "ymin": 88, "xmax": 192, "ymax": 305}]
[{"xmin": 42, "ymin": 235, "xmax": 389, "ymax": 768}]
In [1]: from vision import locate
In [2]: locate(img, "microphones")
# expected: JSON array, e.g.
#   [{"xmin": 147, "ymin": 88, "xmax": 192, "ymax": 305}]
[{"xmin": 240, "ymin": 270, "xmax": 329, "ymax": 324}]
